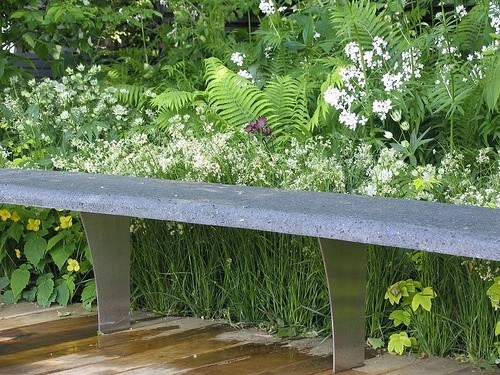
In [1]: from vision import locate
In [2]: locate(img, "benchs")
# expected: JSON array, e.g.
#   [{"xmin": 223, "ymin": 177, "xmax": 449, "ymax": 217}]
[{"xmin": 1, "ymin": 169, "xmax": 500, "ymax": 373}]
